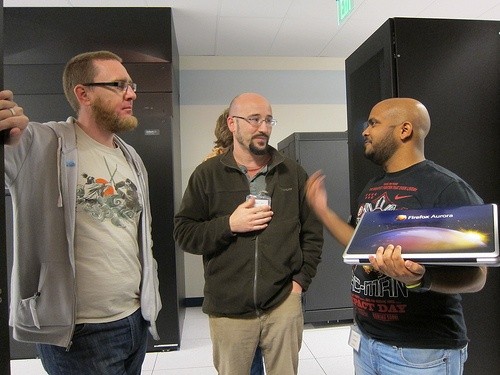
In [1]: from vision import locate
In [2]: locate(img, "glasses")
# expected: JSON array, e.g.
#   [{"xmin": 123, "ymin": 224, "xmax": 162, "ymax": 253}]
[
  {"xmin": 83, "ymin": 81, "xmax": 137, "ymax": 92},
  {"xmin": 232, "ymin": 115, "xmax": 276, "ymax": 128}
]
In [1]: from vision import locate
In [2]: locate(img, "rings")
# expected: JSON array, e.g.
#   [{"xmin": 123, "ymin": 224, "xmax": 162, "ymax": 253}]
[{"xmin": 10, "ymin": 109, "xmax": 15, "ymax": 116}]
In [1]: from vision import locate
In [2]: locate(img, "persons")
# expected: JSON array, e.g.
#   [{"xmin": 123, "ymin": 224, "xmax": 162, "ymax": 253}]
[
  {"xmin": 174, "ymin": 93, "xmax": 324, "ymax": 375},
  {"xmin": 307, "ymin": 98, "xmax": 486, "ymax": 375},
  {"xmin": 0, "ymin": 51, "xmax": 162, "ymax": 375}
]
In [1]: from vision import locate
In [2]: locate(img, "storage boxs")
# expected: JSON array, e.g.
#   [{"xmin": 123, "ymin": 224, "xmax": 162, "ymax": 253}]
[{"xmin": 342, "ymin": 204, "xmax": 500, "ymax": 267}]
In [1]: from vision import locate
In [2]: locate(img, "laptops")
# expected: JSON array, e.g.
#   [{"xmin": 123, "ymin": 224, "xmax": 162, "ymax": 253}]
[{"xmin": 342, "ymin": 203, "xmax": 500, "ymax": 267}]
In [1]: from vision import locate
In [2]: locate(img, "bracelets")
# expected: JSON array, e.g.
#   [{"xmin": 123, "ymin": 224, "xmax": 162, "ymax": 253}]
[{"xmin": 408, "ymin": 268, "xmax": 433, "ymax": 293}]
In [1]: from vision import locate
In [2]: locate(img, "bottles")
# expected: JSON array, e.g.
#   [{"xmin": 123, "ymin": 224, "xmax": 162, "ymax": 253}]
[
  {"xmin": 246, "ymin": 189, "xmax": 257, "ymax": 201},
  {"xmin": 254, "ymin": 190, "xmax": 271, "ymax": 214}
]
